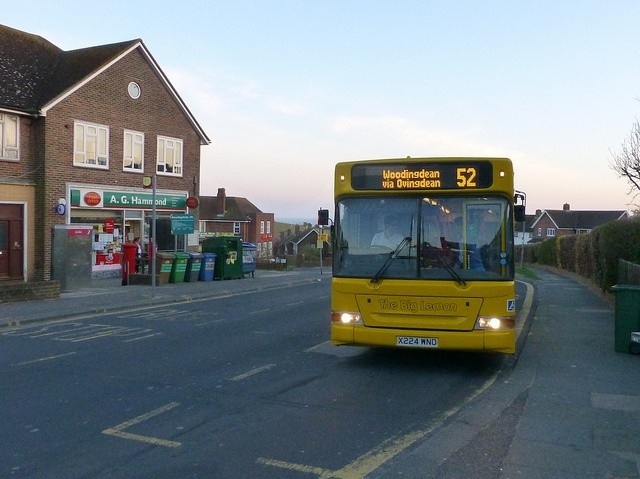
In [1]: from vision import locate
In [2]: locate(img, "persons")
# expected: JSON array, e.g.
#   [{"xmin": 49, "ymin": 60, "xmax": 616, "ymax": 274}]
[
  {"xmin": 127, "ymin": 228, "xmax": 135, "ymax": 243},
  {"xmin": 371, "ymin": 215, "xmax": 408, "ymax": 253},
  {"xmin": 451, "ymin": 217, "xmax": 465, "ymax": 271},
  {"xmin": 133, "ymin": 237, "xmax": 142, "ymax": 273},
  {"xmin": 148, "ymin": 238, "xmax": 158, "ymax": 272}
]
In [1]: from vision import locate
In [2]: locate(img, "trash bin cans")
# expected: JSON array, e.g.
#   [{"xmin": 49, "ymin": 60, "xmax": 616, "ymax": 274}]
[
  {"xmin": 170, "ymin": 252, "xmax": 191, "ymax": 283},
  {"xmin": 241, "ymin": 243, "xmax": 258, "ymax": 278},
  {"xmin": 611, "ymin": 284, "xmax": 639, "ymax": 355},
  {"xmin": 202, "ymin": 236, "xmax": 242, "ymax": 280},
  {"xmin": 184, "ymin": 252, "xmax": 205, "ymax": 282},
  {"xmin": 157, "ymin": 252, "xmax": 176, "ymax": 284},
  {"xmin": 199, "ymin": 252, "xmax": 218, "ymax": 281}
]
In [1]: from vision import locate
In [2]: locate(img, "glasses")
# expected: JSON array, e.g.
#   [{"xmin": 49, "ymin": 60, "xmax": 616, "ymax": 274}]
[{"xmin": 388, "ymin": 223, "xmax": 398, "ymax": 227}]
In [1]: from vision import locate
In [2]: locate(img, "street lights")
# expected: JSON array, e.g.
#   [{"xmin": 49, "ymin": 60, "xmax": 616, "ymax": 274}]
[{"xmin": 516, "ymin": 190, "xmax": 527, "ymax": 270}]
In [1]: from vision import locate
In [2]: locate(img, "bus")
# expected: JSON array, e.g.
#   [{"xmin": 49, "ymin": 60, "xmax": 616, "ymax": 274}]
[{"xmin": 317, "ymin": 155, "xmax": 526, "ymax": 356}]
